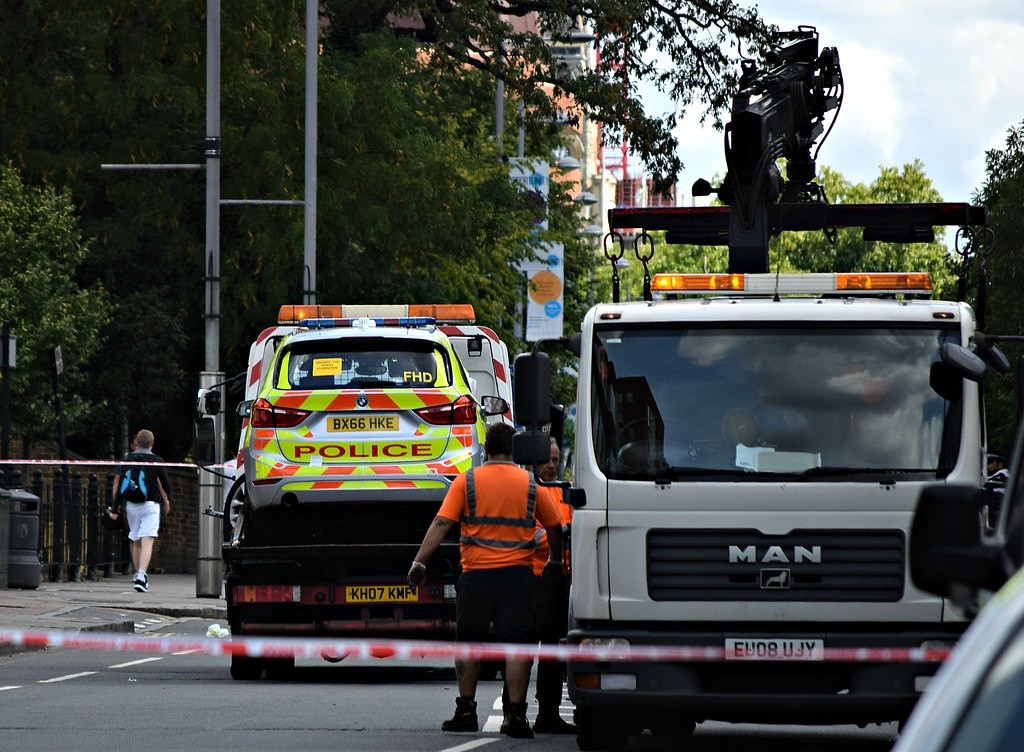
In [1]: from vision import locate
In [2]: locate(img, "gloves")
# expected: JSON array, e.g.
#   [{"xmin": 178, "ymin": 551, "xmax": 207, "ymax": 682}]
[
  {"xmin": 407, "ymin": 562, "xmax": 427, "ymax": 595},
  {"xmin": 542, "ymin": 557, "xmax": 563, "ymax": 584}
]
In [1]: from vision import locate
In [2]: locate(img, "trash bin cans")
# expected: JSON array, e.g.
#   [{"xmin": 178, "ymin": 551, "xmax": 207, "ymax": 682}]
[
  {"xmin": 0, "ymin": 486, "xmax": 12, "ymax": 589},
  {"xmin": 10, "ymin": 488, "xmax": 41, "ymax": 589}
]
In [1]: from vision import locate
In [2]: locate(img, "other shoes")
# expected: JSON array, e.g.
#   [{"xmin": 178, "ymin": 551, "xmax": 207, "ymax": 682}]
[
  {"xmin": 442, "ymin": 694, "xmax": 478, "ymax": 731},
  {"xmin": 533, "ymin": 712, "xmax": 577, "ymax": 736},
  {"xmin": 501, "ymin": 713, "xmax": 509, "ymax": 734},
  {"xmin": 507, "ymin": 703, "xmax": 533, "ymax": 738}
]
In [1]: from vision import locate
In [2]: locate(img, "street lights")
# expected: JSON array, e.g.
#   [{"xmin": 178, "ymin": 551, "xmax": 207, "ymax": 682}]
[{"xmin": 494, "ymin": 31, "xmax": 598, "ymax": 158}]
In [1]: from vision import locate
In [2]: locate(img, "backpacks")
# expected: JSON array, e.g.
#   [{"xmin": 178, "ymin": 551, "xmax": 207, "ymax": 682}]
[{"xmin": 121, "ymin": 451, "xmax": 153, "ymax": 503}]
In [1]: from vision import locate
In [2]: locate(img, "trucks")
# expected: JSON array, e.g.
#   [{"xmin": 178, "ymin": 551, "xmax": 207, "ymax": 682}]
[
  {"xmin": 204, "ymin": 303, "xmax": 516, "ymax": 676},
  {"xmin": 511, "ymin": 25, "xmax": 1024, "ymax": 752}
]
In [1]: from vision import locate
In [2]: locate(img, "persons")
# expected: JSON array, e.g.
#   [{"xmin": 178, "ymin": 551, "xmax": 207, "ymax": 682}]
[
  {"xmin": 408, "ymin": 422, "xmax": 576, "ymax": 738},
  {"xmin": 109, "ymin": 430, "xmax": 170, "ymax": 592}
]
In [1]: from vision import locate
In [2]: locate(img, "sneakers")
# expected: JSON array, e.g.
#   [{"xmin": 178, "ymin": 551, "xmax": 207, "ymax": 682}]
[{"xmin": 134, "ymin": 571, "xmax": 148, "ymax": 593}]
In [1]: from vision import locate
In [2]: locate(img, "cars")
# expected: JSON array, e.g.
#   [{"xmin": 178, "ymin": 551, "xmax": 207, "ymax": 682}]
[{"xmin": 236, "ymin": 316, "xmax": 511, "ymax": 535}]
